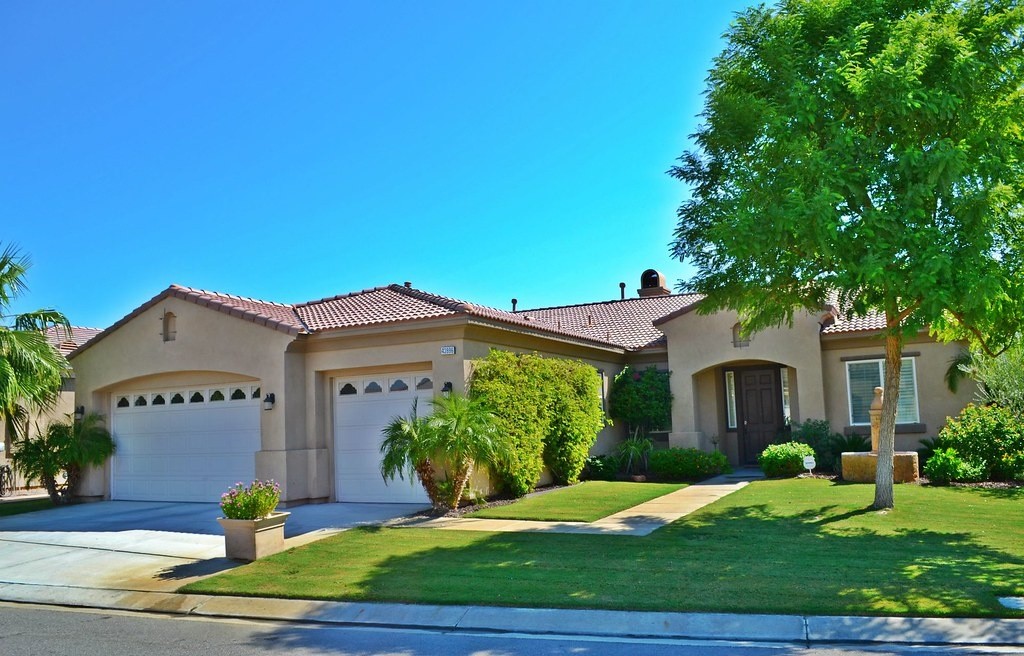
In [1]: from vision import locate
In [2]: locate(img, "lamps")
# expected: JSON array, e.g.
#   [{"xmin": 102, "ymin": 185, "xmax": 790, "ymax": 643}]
[
  {"xmin": 441, "ymin": 381, "xmax": 452, "ymax": 400},
  {"xmin": 263, "ymin": 393, "xmax": 275, "ymax": 410},
  {"xmin": 74, "ymin": 405, "xmax": 85, "ymax": 420}
]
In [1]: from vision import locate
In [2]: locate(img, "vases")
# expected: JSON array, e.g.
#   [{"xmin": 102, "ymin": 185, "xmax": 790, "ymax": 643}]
[{"xmin": 216, "ymin": 512, "xmax": 292, "ymax": 561}]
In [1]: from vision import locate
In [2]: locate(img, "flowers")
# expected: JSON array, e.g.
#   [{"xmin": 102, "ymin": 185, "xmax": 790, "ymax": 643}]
[{"xmin": 216, "ymin": 478, "xmax": 291, "ymax": 520}]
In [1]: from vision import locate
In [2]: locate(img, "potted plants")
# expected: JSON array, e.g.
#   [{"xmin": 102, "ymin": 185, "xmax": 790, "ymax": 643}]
[{"xmin": 617, "ymin": 437, "xmax": 652, "ymax": 482}]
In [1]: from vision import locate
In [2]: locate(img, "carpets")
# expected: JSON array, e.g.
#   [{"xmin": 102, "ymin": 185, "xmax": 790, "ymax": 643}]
[{"xmin": 726, "ymin": 467, "xmax": 766, "ymax": 479}]
